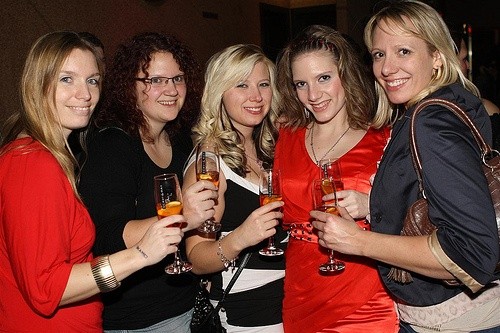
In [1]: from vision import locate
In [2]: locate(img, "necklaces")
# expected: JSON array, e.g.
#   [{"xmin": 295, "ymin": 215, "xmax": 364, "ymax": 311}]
[
  {"xmin": 245, "ymin": 154, "xmax": 264, "ymax": 178},
  {"xmin": 310, "ymin": 123, "xmax": 351, "ymax": 166}
]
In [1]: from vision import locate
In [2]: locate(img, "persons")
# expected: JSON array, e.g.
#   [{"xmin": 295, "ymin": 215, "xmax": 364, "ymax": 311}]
[{"xmin": 0, "ymin": 1, "xmax": 500, "ymax": 333}]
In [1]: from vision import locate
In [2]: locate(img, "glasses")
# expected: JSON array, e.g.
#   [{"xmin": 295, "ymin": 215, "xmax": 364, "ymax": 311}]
[{"xmin": 135, "ymin": 75, "xmax": 188, "ymax": 86}]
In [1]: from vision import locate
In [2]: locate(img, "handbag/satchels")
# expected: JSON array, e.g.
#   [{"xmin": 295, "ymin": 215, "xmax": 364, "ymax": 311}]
[
  {"xmin": 387, "ymin": 98, "xmax": 500, "ymax": 286},
  {"xmin": 190, "ymin": 288, "xmax": 226, "ymax": 333}
]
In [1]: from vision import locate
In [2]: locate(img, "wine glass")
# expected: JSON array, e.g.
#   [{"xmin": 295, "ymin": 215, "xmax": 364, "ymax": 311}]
[
  {"xmin": 312, "ymin": 178, "xmax": 346, "ymax": 271},
  {"xmin": 196, "ymin": 140, "xmax": 221, "ymax": 234},
  {"xmin": 154, "ymin": 174, "xmax": 193, "ymax": 274},
  {"xmin": 259, "ymin": 169, "xmax": 284, "ymax": 256},
  {"xmin": 319, "ymin": 158, "xmax": 344, "ymax": 202}
]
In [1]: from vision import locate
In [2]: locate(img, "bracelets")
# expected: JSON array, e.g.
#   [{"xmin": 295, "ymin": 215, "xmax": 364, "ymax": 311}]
[
  {"xmin": 216, "ymin": 235, "xmax": 238, "ymax": 268},
  {"xmin": 91, "ymin": 254, "xmax": 121, "ymax": 293}
]
